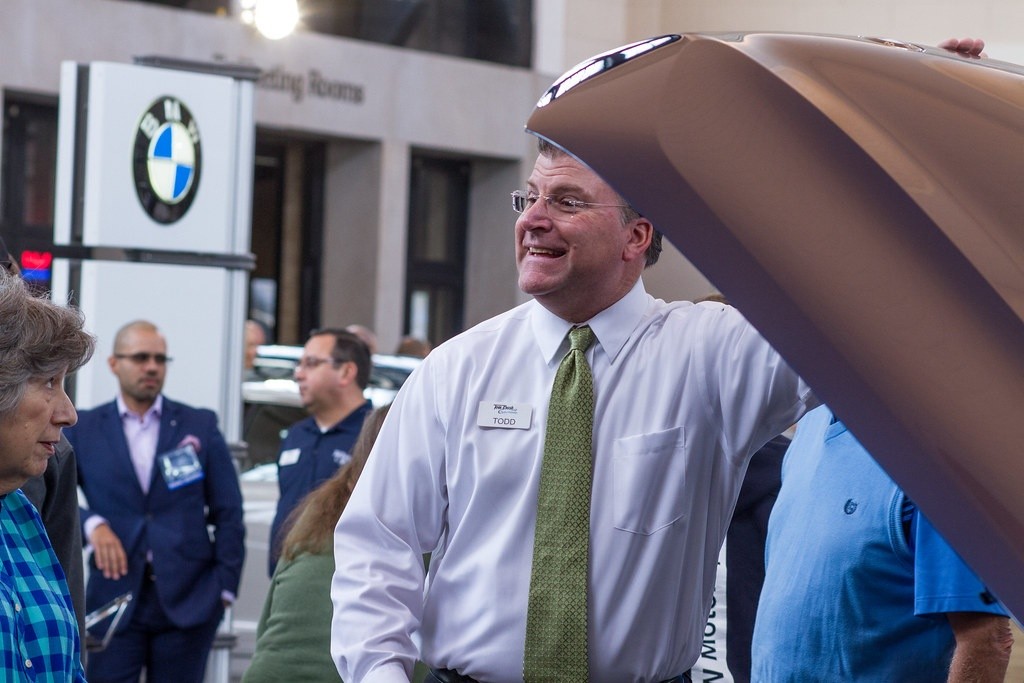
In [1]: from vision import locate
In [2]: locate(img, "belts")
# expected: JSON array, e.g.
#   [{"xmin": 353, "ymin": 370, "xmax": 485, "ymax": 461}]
[{"xmin": 431, "ymin": 668, "xmax": 479, "ymax": 683}]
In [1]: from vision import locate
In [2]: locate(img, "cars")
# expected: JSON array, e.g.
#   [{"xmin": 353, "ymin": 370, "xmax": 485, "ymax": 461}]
[{"xmin": 230, "ymin": 342, "xmax": 422, "ymax": 683}]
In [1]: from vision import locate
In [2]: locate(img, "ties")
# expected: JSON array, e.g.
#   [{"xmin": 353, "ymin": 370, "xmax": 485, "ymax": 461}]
[{"xmin": 522, "ymin": 328, "xmax": 593, "ymax": 683}]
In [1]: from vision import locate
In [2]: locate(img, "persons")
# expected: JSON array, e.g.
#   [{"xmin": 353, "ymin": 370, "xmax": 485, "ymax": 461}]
[
  {"xmin": 329, "ymin": 38, "xmax": 989, "ymax": 683},
  {"xmin": 0, "ymin": 254, "xmax": 97, "ymax": 683},
  {"xmin": 61, "ymin": 321, "xmax": 247, "ymax": 683},
  {"xmin": 689, "ymin": 300, "xmax": 1014, "ymax": 682},
  {"xmin": 242, "ymin": 327, "xmax": 431, "ymax": 683}
]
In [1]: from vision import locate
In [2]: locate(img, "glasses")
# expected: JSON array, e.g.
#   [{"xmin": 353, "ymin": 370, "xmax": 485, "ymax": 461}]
[
  {"xmin": 508, "ymin": 188, "xmax": 625, "ymax": 222},
  {"xmin": 116, "ymin": 352, "xmax": 170, "ymax": 364},
  {"xmin": 293, "ymin": 356, "xmax": 328, "ymax": 368}
]
